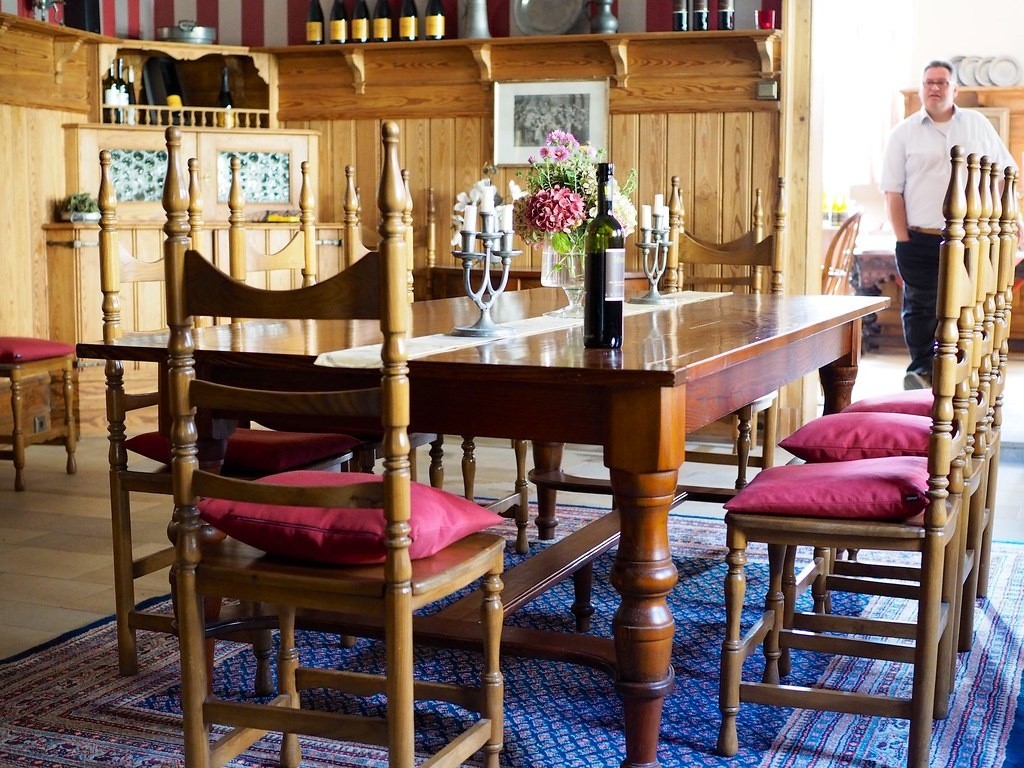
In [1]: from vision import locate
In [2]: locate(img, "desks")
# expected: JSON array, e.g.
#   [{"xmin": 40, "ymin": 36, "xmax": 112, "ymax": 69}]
[{"xmin": 76, "ymin": 287, "xmax": 891, "ymax": 767}]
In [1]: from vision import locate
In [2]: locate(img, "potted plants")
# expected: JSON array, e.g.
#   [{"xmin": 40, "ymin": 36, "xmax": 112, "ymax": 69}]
[{"xmin": 55, "ymin": 192, "xmax": 102, "ymax": 221}]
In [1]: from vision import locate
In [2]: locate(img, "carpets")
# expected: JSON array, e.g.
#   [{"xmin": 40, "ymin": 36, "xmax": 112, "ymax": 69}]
[{"xmin": 0, "ymin": 495, "xmax": 1024, "ymax": 768}]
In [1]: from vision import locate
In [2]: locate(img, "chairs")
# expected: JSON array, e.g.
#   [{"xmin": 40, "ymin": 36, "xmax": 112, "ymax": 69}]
[
  {"xmin": 0, "ymin": 352, "xmax": 78, "ymax": 492},
  {"xmin": 101, "ymin": 121, "xmax": 1018, "ymax": 767}
]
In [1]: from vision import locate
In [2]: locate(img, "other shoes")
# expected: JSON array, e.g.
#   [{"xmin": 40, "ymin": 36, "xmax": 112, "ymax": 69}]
[{"xmin": 904, "ymin": 371, "xmax": 932, "ymax": 389}]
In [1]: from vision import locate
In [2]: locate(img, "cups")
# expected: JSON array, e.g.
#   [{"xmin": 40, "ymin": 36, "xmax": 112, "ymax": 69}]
[{"xmin": 755, "ymin": 10, "xmax": 775, "ymax": 30}]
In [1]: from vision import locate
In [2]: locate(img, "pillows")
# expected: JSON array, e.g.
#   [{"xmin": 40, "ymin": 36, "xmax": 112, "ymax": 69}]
[
  {"xmin": 0, "ymin": 336, "xmax": 76, "ymax": 363},
  {"xmin": 840, "ymin": 388, "xmax": 934, "ymax": 417},
  {"xmin": 197, "ymin": 470, "xmax": 506, "ymax": 563},
  {"xmin": 722, "ymin": 456, "xmax": 930, "ymax": 522},
  {"xmin": 776, "ymin": 412, "xmax": 933, "ymax": 464},
  {"xmin": 120, "ymin": 428, "xmax": 362, "ymax": 479}
]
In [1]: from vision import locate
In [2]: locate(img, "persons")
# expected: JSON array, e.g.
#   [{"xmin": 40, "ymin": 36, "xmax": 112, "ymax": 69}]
[{"xmin": 883, "ymin": 60, "xmax": 1019, "ymax": 390}]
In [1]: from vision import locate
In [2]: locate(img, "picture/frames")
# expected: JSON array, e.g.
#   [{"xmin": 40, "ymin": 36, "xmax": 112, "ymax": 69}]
[
  {"xmin": 967, "ymin": 106, "xmax": 1011, "ymax": 153},
  {"xmin": 495, "ymin": 78, "xmax": 613, "ymax": 167}
]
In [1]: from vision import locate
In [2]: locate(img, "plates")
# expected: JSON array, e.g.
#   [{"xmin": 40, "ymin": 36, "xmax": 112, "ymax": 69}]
[{"xmin": 513, "ymin": 0, "xmax": 583, "ymax": 36}]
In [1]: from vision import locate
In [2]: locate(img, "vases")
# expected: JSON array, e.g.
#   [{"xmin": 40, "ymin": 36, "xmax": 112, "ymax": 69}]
[{"xmin": 541, "ymin": 234, "xmax": 586, "ymax": 319}]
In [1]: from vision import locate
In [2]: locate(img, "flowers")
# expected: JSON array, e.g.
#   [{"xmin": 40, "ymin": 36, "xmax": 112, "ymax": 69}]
[
  {"xmin": 509, "ymin": 129, "xmax": 640, "ymax": 281},
  {"xmin": 450, "ymin": 177, "xmax": 529, "ymax": 249}
]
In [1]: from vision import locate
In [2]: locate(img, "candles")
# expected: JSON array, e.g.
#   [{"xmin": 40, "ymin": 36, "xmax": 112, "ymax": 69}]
[
  {"xmin": 654, "ymin": 194, "xmax": 664, "ymax": 214},
  {"xmin": 479, "ymin": 185, "xmax": 494, "ymax": 214},
  {"xmin": 499, "ymin": 205, "xmax": 514, "ymax": 232},
  {"xmin": 641, "ymin": 205, "xmax": 652, "ymax": 231},
  {"xmin": 463, "ymin": 204, "xmax": 478, "ymax": 233},
  {"xmin": 661, "ymin": 206, "xmax": 670, "ymax": 230}
]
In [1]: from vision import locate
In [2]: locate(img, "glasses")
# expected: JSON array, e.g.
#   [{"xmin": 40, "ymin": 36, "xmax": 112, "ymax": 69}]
[{"xmin": 924, "ymin": 78, "xmax": 949, "ymax": 89}]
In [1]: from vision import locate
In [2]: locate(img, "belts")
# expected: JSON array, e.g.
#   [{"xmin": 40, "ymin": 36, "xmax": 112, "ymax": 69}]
[{"xmin": 910, "ymin": 226, "xmax": 943, "ymax": 235}]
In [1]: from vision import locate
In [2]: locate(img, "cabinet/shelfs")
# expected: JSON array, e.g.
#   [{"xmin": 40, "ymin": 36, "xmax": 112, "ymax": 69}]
[
  {"xmin": 42, "ymin": 44, "xmax": 347, "ymax": 442},
  {"xmin": 854, "ymin": 86, "xmax": 1024, "ymax": 351}
]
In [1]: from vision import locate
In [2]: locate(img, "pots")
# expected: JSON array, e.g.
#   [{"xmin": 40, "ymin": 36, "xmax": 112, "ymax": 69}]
[{"xmin": 157, "ymin": 20, "xmax": 216, "ymax": 44}]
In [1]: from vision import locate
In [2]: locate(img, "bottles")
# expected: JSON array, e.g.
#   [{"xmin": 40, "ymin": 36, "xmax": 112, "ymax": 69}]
[
  {"xmin": 398, "ymin": 0, "xmax": 419, "ymax": 41},
  {"xmin": 693, "ymin": 0, "xmax": 708, "ymax": 31},
  {"xmin": 673, "ymin": 0, "xmax": 688, "ymax": 31},
  {"xmin": 351, "ymin": 0, "xmax": 370, "ymax": 43},
  {"xmin": 305, "ymin": 0, "xmax": 324, "ymax": 45},
  {"xmin": 102, "ymin": 58, "xmax": 136, "ymax": 124},
  {"xmin": 584, "ymin": 163, "xmax": 625, "ymax": 348},
  {"xmin": 372, "ymin": 0, "xmax": 392, "ymax": 42},
  {"xmin": 424, "ymin": 0, "xmax": 445, "ymax": 40},
  {"xmin": 215, "ymin": 67, "xmax": 235, "ymax": 127},
  {"xmin": 718, "ymin": 0, "xmax": 734, "ymax": 30},
  {"xmin": 329, "ymin": 0, "xmax": 348, "ymax": 44},
  {"xmin": 163, "ymin": 63, "xmax": 183, "ymax": 117}
]
